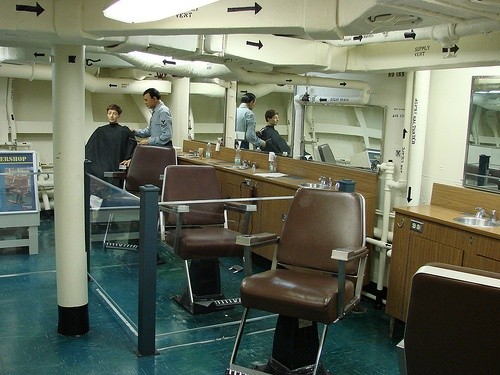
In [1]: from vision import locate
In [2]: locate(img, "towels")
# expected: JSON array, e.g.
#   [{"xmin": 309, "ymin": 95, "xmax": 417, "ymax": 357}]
[{"xmin": 253, "ymin": 172, "xmax": 289, "ymax": 178}]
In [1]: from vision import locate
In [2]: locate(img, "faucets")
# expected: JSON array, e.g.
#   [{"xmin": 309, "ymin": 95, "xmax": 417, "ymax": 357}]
[
  {"xmin": 190, "ymin": 150, "xmax": 195, "ymax": 155},
  {"xmin": 324, "ymin": 180, "xmax": 333, "ymax": 187},
  {"xmin": 271, "ymin": 161, "xmax": 277, "ymax": 171},
  {"xmin": 474, "ymin": 206, "xmax": 496, "ymax": 221},
  {"xmin": 243, "ymin": 160, "xmax": 256, "ymax": 168}
]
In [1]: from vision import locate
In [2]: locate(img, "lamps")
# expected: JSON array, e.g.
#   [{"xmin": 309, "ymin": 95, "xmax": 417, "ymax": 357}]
[{"xmin": 102, "ymin": 0, "xmax": 220, "ymax": 24}]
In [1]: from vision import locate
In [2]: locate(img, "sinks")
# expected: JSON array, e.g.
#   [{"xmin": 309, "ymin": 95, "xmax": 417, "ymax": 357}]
[
  {"xmin": 298, "ymin": 182, "xmax": 331, "ymax": 190},
  {"xmin": 221, "ymin": 164, "xmax": 251, "ymax": 170},
  {"xmin": 178, "ymin": 154, "xmax": 199, "ymax": 158},
  {"xmin": 453, "ymin": 216, "xmax": 500, "ymax": 227}
]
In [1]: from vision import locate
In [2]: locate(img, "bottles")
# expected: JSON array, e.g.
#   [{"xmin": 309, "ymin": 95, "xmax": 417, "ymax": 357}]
[
  {"xmin": 235, "ymin": 147, "xmax": 242, "ymax": 165},
  {"xmin": 206, "ymin": 143, "xmax": 212, "ymax": 158},
  {"xmin": 269, "ymin": 161, "xmax": 278, "ymax": 173}
]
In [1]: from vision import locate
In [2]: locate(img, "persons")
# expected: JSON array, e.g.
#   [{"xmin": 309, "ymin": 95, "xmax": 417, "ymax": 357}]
[
  {"xmin": 258, "ymin": 110, "xmax": 291, "ymax": 156},
  {"xmin": 234, "ymin": 92, "xmax": 266, "ymax": 149},
  {"xmin": 131, "ymin": 88, "xmax": 173, "ymax": 147},
  {"xmin": 85, "ymin": 105, "xmax": 138, "ymax": 168}
]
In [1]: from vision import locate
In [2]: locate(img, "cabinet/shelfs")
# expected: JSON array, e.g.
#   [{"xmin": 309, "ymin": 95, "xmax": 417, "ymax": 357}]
[
  {"xmin": 177, "ymin": 158, "xmax": 380, "ymax": 288},
  {"xmin": 385, "ymin": 211, "xmax": 500, "ymax": 339}
]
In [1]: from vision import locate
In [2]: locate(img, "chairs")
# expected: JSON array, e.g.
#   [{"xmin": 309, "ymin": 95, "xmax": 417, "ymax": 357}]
[
  {"xmin": 225, "ymin": 186, "xmax": 370, "ymax": 375},
  {"xmin": 103, "ymin": 144, "xmax": 177, "ymax": 265},
  {"xmin": 160, "ymin": 164, "xmax": 257, "ymax": 314},
  {"xmin": 403, "ymin": 261, "xmax": 500, "ymax": 375}
]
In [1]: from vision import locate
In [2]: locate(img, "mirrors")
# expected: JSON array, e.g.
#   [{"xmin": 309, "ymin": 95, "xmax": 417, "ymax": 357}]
[{"xmin": 461, "ymin": 75, "xmax": 500, "ymax": 194}]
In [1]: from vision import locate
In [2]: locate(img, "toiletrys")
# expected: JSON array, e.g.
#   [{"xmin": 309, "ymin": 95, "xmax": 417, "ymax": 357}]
[
  {"xmin": 270, "ymin": 153, "xmax": 275, "ymax": 172},
  {"xmin": 205, "ymin": 142, "xmax": 211, "ymax": 158},
  {"xmin": 235, "ymin": 147, "xmax": 241, "ymax": 164}
]
